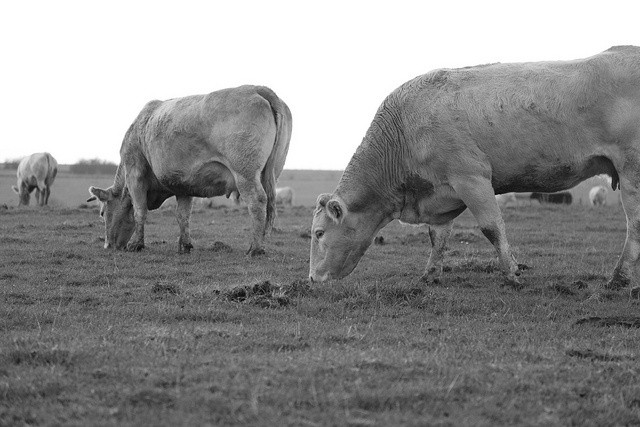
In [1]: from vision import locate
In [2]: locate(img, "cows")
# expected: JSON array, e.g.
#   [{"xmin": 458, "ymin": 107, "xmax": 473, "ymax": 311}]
[
  {"xmin": 308, "ymin": 44, "xmax": 639, "ymax": 298},
  {"xmin": 589, "ymin": 185, "xmax": 608, "ymax": 207},
  {"xmin": 494, "ymin": 191, "xmax": 517, "ymax": 205},
  {"xmin": 528, "ymin": 191, "xmax": 572, "ymax": 205},
  {"xmin": 274, "ymin": 186, "xmax": 292, "ymax": 207},
  {"xmin": 11, "ymin": 151, "xmax": 58, "ymax": 208},
  {"xmin": 86, "ymin": 83, "xmax": 293, "ymax": 263}
]
